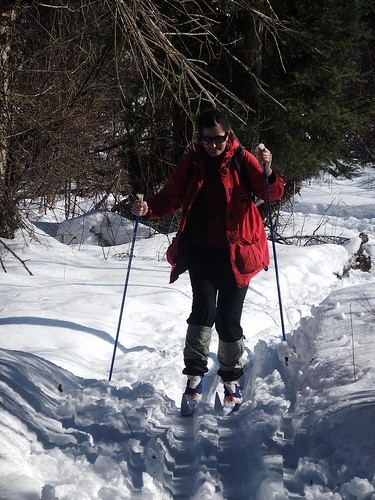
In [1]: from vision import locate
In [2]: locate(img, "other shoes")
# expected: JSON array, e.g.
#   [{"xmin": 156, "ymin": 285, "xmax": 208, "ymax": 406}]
[
  {"xmin": 185, "ymin": 374, "xmax": 203, "ymax": 405},
  {"xmin": 224, "ymin": 380, "xmax": 244, "ymax": 405}
]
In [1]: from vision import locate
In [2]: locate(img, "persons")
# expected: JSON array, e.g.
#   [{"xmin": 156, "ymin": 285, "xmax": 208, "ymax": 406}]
[{"xmin": 130, "ymin": 111, "xmax": 284, "ymax": 417}]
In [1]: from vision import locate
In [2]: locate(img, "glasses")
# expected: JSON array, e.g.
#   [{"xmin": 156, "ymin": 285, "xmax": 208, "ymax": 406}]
[{"xmin": 201, "ymin": 131, "xmax": 228, "ymax": 145}]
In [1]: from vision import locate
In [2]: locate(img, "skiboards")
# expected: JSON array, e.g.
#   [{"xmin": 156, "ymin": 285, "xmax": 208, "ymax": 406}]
[{"xmin": 180, "ymin": 392, "xmax": 240, "ymax": 417}]
